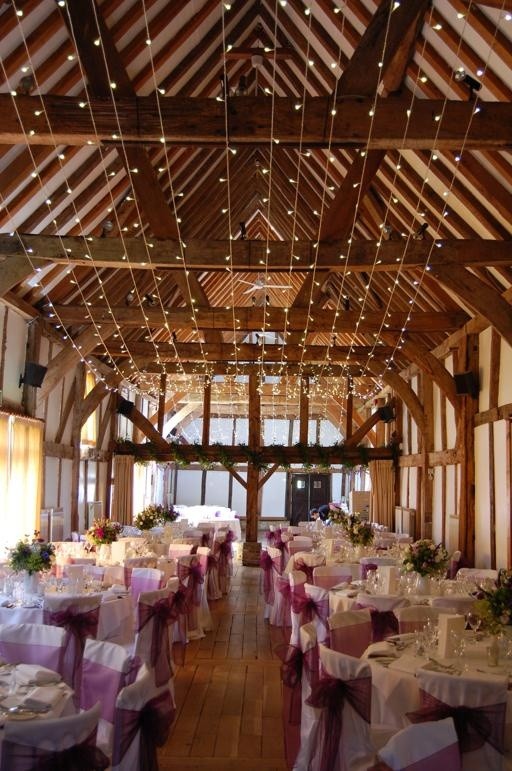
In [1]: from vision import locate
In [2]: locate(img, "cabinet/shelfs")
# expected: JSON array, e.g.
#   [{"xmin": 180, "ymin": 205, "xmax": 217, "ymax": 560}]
[{"xmin": 349, "ymin": 490, "xmax": 370, "ymax": 520}]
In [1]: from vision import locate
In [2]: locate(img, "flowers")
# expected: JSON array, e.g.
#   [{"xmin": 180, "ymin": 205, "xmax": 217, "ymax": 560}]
[
  {"xmin": 133, "ymin": 502, "xmax": 179, "ymax": 530},
  {"xmin": 327, "ymin": 506, "xmax": 349, "ymax": 523}
]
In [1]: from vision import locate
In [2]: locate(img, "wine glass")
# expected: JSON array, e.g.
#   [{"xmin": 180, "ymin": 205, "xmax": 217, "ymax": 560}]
[
  {"xmin": 0, "ymin": 525, "xmax": 203, "ymax": 719},
  {"xmin": 397, "ymin": 609, "xmax": 509, "ymax": 680},
  {"xmin": 306, "ymin": 519, "xmax": 478, "ymax": 598}
]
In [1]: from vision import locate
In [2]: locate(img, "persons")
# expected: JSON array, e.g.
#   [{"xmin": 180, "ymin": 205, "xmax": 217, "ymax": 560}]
[{"xmin": 309, "ymin": 503, "xmax": 343, "ymax": 525}]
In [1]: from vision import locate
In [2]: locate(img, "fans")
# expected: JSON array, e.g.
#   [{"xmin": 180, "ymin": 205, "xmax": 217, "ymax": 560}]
[{"xmin": 236, "ymin": 272, "xmax": 293, "ymax": 294}]
